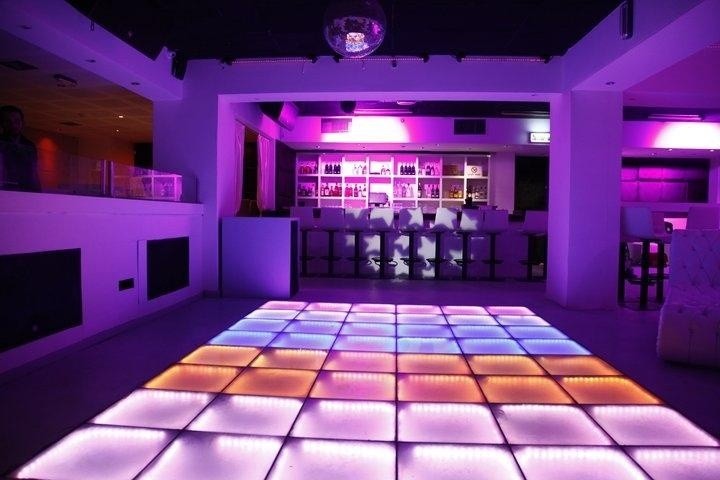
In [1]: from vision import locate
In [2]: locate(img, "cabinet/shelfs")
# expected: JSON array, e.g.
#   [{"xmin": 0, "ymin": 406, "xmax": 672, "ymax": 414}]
[
  {"xmin": 397, "ymin": 181, "xmax": 488, "ymax": 198},
  {"xmin": 297, "ymin": 181, "xmax": 366, "ymax": 198},
  {"xmin": 299, "ymin": 161, "xmax": 435, "ymax": 176}
]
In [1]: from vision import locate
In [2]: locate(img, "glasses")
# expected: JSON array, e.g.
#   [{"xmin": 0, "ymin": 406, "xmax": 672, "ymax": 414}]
[{"xmin": 324, "ymin": 0, "xmax": 385, "ymax": 59}]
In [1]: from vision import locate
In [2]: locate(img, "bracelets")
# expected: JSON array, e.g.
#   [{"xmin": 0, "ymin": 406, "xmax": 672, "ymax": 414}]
[{"xmin": 461, "ymin": 205, "xmax": 479, "ymax": 210}]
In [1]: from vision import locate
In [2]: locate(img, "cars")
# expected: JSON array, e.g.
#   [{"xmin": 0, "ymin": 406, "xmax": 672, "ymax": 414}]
[{"xmin": 465, "ymin": 197, "xmax": 472, "ymax": 205}]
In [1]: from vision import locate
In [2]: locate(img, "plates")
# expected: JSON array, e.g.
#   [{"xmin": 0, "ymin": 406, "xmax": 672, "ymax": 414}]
[{"xmin": 294, "ymin": 152, "xmax": 491, "ymax": 222}]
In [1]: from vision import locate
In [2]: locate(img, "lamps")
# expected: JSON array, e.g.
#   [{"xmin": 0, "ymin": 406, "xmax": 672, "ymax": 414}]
[
  {"xmin": 296, "ymin": 206, "xmax": 549, "ymax": 283},
  {"xmin": 618, "ymin": 206, "xmax": 665, "ymax": 308},
  {"xmin": 656, "ymin": 229, "xmax": 720, "ymax": 369}
]
